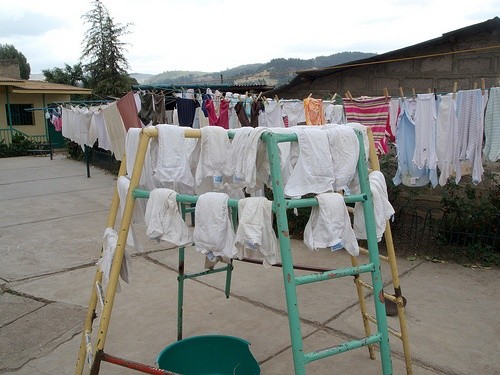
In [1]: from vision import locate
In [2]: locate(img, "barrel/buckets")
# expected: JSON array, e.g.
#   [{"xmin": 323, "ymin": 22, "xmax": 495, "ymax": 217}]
[{"xmin": 155, "ymin": 333, "xmax": 261, "ymax": 375}]
